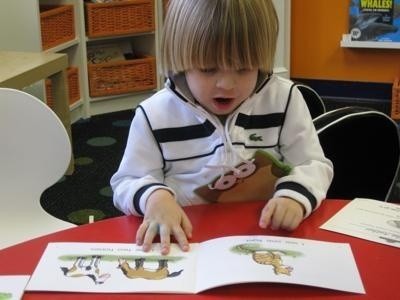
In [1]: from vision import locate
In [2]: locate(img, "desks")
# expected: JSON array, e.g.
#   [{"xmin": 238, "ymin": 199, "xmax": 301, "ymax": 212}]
[
  {"xmin": 1, "ymin": 195, "xmax": 400, "ymax": 300},
  {"xmin": 0, "ymin": 48, "xmax": 75, "ymax": 177}
]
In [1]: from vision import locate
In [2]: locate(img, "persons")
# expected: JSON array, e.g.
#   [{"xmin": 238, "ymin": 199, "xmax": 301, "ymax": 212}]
[{"xmin": 109, "ymin": 0, "xmax": 335, "ymax": 255}]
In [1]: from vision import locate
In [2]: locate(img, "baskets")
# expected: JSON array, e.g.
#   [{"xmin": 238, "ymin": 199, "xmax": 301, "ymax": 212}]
[
  {"xmin": 85, "ymin": 0, "xmax": 156, "ymax": 39},
  {"xmin": 39, "ymin": 3, "xmax": 78, "ymax": 52},
  {"xmin": 45, "ymin": 65, "xmax": 81, "ymax": 112},
  {"xmin": 86, "ymin": 49, "xmax": 158, "ymax": 98}
]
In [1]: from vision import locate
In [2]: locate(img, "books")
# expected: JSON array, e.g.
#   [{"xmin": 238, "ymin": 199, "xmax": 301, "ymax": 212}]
[
  {"xmin": 24, "ymin": 234, "xmax": 366, "ymax": 295},
  {"xmin": 86, "ymin": 43, "xmax": 126, "ymax": 64}
]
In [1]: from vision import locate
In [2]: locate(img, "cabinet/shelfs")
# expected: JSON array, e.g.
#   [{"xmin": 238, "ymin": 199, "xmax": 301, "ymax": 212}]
[{"xmin": 0, "ymin": 1, "xmax": 293, "ymax": 133}]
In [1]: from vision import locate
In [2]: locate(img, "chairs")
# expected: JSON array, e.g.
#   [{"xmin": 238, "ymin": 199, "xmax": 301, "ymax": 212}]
[{"xmin": 0, "ymin": 89, "xmax": 79, "ymax": 249}]
